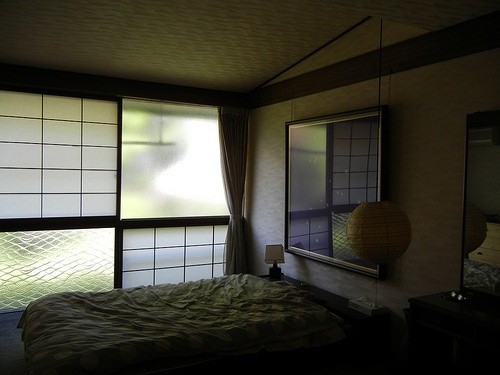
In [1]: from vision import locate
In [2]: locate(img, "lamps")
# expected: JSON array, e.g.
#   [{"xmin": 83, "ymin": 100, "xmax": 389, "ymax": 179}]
[
  {"xmin": 464, "ymin": 200, "xmax": 488, "ymax": 254},
  {"xmin": 346, "ymin": 17, "xmax": 413, "ymax": 265},
  {"xmin": 264, "ymin": 243, "xmax": 285, "ymax": 282}
]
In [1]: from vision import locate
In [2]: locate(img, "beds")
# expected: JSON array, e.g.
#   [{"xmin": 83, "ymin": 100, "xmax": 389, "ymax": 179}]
[{"xmin": 16, "ymin": 273, "xmax": 352, "ymax": 375}]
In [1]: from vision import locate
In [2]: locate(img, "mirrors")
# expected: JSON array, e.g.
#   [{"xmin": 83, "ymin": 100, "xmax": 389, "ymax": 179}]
[{"xmin": 460, "ymin": 110, "xmax": 500, "ymax": 312}]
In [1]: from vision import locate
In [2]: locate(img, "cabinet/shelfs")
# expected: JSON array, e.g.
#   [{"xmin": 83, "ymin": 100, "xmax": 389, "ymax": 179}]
[{"xmin": 402, "ymin": 290, "xmax": 500, "ymax": 375}]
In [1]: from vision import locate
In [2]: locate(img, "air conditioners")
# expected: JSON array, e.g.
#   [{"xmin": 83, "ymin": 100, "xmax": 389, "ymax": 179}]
[{"xmin": 469, "ymin": 126, "xmax": 497, "ymax": 147}]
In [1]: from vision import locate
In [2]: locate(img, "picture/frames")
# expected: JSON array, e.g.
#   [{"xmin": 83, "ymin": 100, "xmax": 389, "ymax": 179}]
[{"xmin": 283, "ymin": 102, "xmax": 388, "ymax": 284}]
